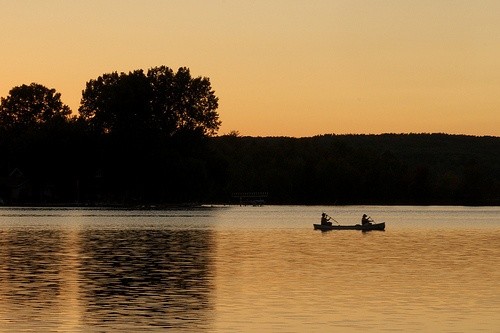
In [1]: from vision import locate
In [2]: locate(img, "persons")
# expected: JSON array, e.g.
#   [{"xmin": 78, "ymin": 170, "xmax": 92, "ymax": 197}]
[
  {"xmin": 362, "ymin": 214, "xmax": 373, "ymax": 225},
  {"xmin": 321, "ymin": 212, "xmax": 332, "ymax": 225}
]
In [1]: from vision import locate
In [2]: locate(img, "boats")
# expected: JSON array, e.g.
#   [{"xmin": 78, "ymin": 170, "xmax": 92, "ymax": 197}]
[
  {"xmin": 314, "ymin": 223, "xmax": 361, "ymax": 230},
  {"xmin": 355, "ymin": 222, "xmax": 385, "ymax": 230}
]
